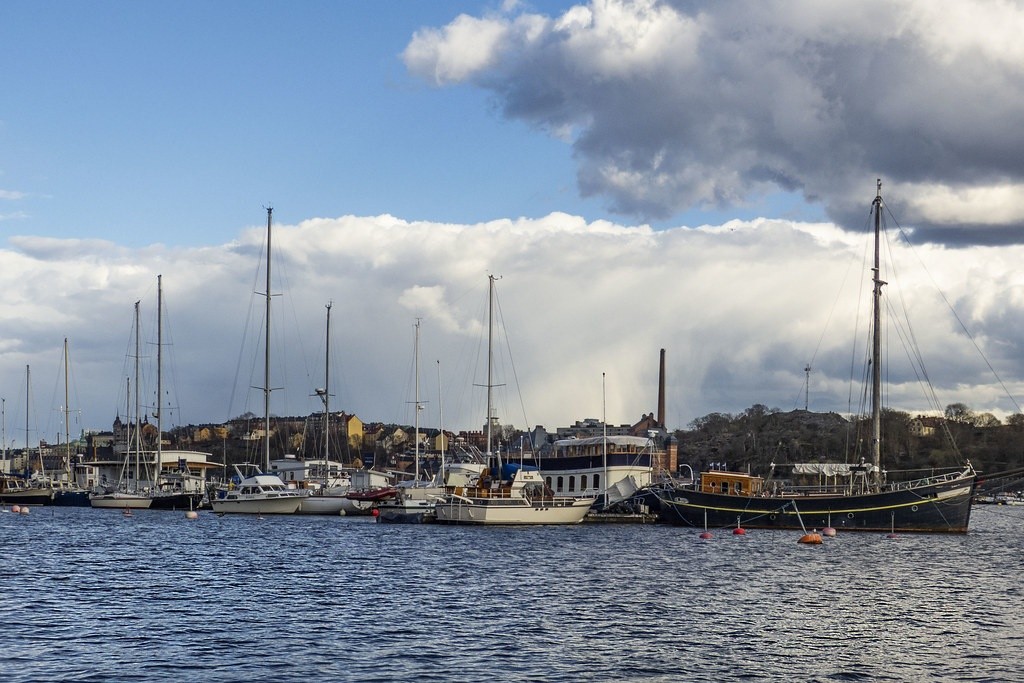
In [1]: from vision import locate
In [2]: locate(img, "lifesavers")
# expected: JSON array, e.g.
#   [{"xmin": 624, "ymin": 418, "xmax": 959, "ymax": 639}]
[
  {"xmin": 911, "ymin": 504, "xmax": 918, "ymax": 512},
  {"xmin": 770, "ymin": 514, "xmax": 776, "ymax": 521},
  {"xmin": 847, "ymin": 512, "xmax": 855, "ymax": 520}
]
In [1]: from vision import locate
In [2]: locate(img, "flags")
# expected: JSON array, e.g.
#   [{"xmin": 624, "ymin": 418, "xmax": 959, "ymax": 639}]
[
  {"xmin": 722, "ymin": 463, "xmax": 726, "ymax": 466},
  {"xmin": 710, "ymin": 462, "xmax": 713, "ymax": 466},
  {"xmin": 717, "ymin": 462, "xmax": 719, "ymax": 466}
]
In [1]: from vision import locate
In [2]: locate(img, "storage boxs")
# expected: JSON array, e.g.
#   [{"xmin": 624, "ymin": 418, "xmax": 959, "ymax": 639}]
[
  {"xmin": 467, "ymin": 487, "xmax": 487, "ymax": 497},
  {"xmin": 455, "ymin": 487, "xmax": 468, "ymax": 496},
  {"xmin": 492, "ymin": 488, "xmax": 510, "ymax": 498}
]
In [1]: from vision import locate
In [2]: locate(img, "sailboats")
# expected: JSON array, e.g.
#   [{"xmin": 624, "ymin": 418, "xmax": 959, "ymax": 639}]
[
  {"xmin": 645, "ymin": 173, "xmax": 1024, "ymax": 537},
  {"xmin": 434, "ymin": 271, "xmax": 602, "ymax": 526},
  {"xmin": 0, "ymin": 201, "xmax": 698, "ymax": 524}
]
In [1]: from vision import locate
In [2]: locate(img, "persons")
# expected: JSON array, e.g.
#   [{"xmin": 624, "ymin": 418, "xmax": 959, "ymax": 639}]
[{"xmin": 228, "ymin": 481, "xmax": 236, "ymax": 491}]
